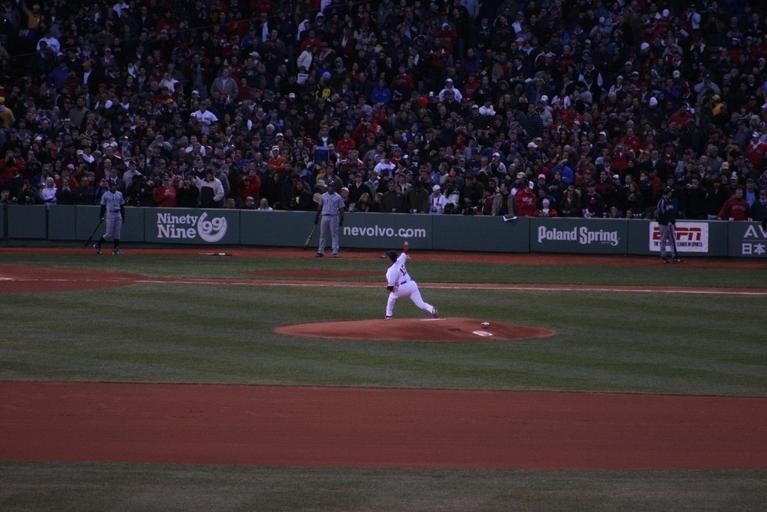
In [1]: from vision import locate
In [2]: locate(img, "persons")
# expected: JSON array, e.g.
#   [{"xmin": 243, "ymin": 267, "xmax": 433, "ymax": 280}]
[
  {"xmin": 0, "ymin": 0, "xmax": 765, "ymax": 227},
  {"xmin": 381, "ymin": 240, "xmax": 438, "ymax": 319},
  {"xmin": 313, "ymin": 179, "xmax": 347, "ymax": 258},
  {"xmin": 656, "ymin": 185, "xmax": 686, "ymax": 264},
  {"xmin": 92, "ymin": 181, "xmax": 128, "ymax": 257}
]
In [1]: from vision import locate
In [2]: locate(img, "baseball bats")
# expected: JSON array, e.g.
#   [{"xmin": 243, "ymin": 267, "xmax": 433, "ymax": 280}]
[
  {"xmin": 83, "ymin": 219, "xmax": 101, "ymax": 248},
  {"xmin": 200, "ymin": 252, "xmax": 232, "ymax": 256},
  {"xmin": 303, "ymin": 226, "xmax": 315, "ymax": 249}
]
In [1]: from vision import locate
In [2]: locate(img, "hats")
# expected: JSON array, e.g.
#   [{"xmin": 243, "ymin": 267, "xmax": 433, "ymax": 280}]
[
  {"xmin": 386, "ymin": 251, "xmax": 397, "ymax": 262},
  {"xmin": 328, "ymin": 181, "xmax": 336, "ymax": 186},
  {"xmin": 0, "ymin": 4, "xmax": 766, "ymax": 192}
]
